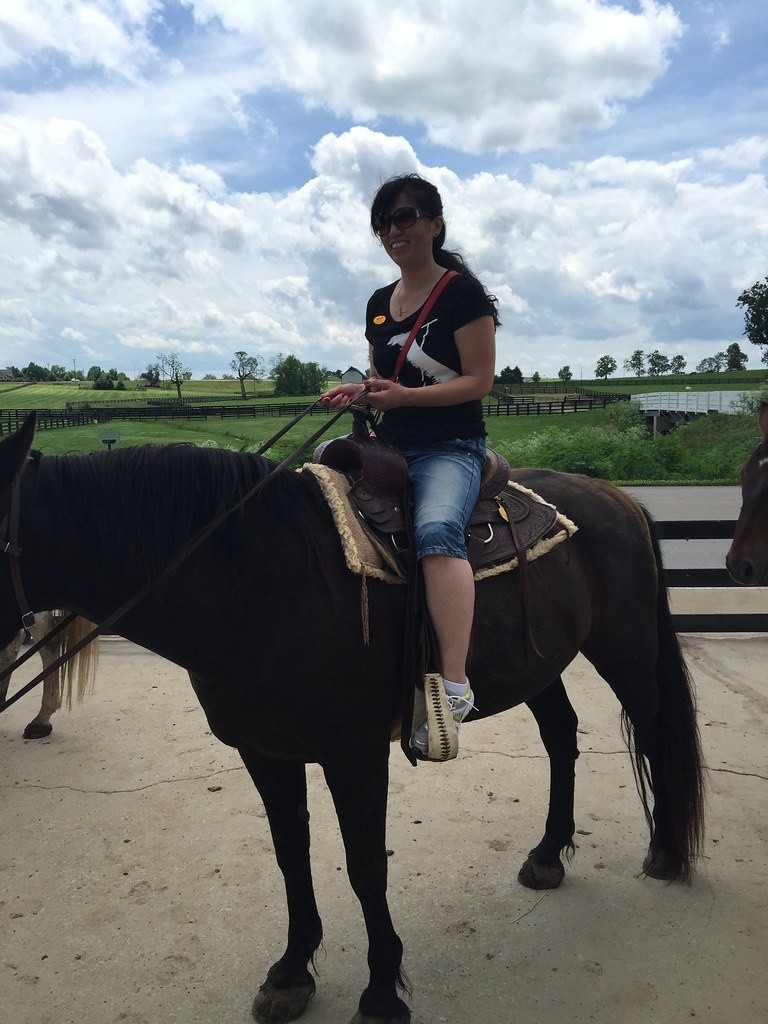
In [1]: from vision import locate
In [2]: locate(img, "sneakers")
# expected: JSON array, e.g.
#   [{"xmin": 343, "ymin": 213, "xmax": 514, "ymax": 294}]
[{"xmin": 408, "ymin": 689, "xmax": 480, "ymax": 756}]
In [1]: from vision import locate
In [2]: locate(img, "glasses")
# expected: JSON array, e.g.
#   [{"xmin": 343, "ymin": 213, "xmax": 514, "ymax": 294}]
[{"xmin": 375, "ymin": 206, "xmax": 435, "ymax": 236}]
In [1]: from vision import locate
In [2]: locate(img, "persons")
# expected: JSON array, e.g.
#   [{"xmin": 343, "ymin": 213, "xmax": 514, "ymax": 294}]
[{"xmin": 316, "ymin": 173, "xmax": 503, "ymax": 755}]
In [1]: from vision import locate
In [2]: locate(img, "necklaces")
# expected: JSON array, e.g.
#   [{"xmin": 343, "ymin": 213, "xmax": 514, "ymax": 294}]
[{"xmin": 397, "ymin": 270, "xmax": 446, "ymax": 317}]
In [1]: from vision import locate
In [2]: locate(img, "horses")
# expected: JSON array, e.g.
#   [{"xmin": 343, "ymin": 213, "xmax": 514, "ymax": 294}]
[
  {"xmin": 724, "ymin": 400, "xmax": 768, "ymax": 588},
  {"xmin": 0, "ymin": 409, "xmax": 703, "ymax": 1023}
]
